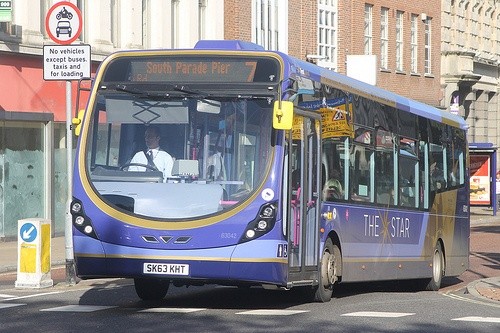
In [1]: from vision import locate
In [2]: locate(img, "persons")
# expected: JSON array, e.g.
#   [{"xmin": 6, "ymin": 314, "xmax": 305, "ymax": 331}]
[
  {"xmin": 328, "ymin": 163, "xmax": 344, "ymax": 191},
  {"xmin": 322, "ymin": 178, "xmax": 343, "ymax": 201},
  {"xmin": 127, "ymin": 125, "xmax": 174, "ymax": 183}
]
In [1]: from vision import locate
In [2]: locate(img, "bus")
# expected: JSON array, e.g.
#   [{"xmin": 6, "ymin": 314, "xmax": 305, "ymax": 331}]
[{"xmin": 70, "ymin": 39, "xmax": 470, "ymax": 303}]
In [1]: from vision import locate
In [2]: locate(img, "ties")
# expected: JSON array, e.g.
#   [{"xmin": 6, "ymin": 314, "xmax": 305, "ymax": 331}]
[{"xmin": 147, "ymin": 151, "xmax": 153, "ymax": 172}]
